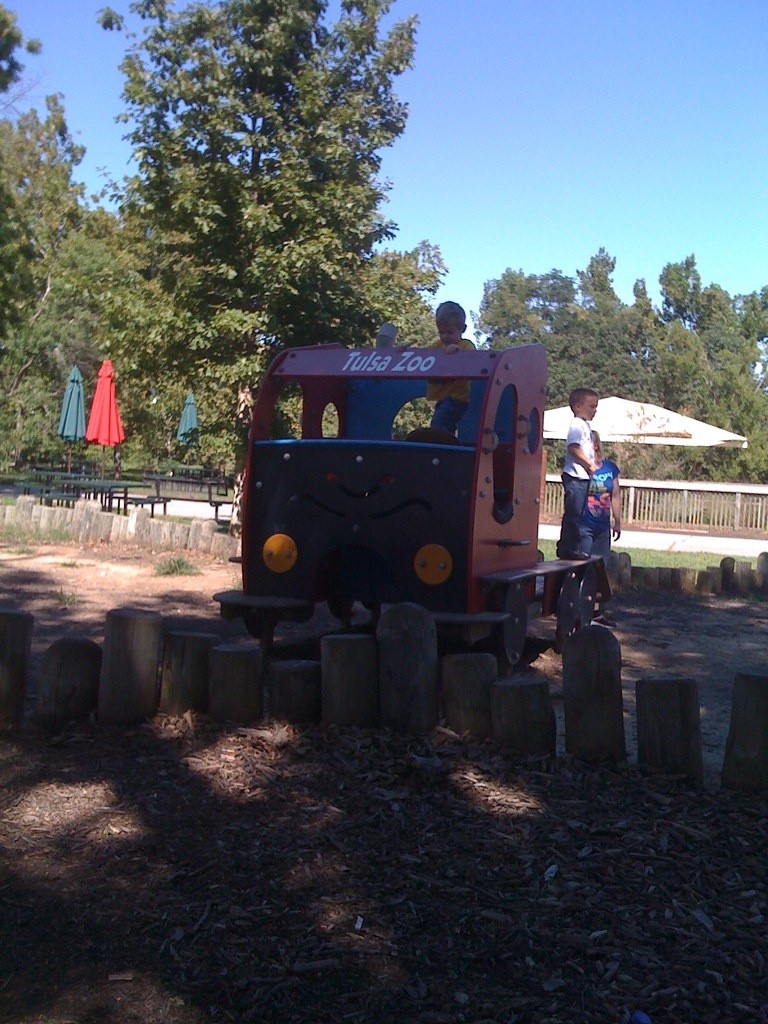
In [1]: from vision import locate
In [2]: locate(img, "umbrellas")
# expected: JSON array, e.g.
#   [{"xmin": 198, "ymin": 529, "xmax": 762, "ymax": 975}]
[
  {"xmin": 177, "ymin": 395, "xmax": 197, "ymax": 447},
  {"xmin": 543, "ymin": 397, "xmax": 748, "ymax": 448},
  {"xmin": 57, "ymin": 366, "xmax": 86, "ymax": 473},
  {"xmin": 85, "ymin": 360, "xmax": 125, "ymax": 480}
]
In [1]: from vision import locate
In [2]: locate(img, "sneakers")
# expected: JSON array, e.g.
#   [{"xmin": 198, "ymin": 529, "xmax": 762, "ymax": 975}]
[
  {"xmin": 556, "ymin": 549, "xmax": 591, "ymax": 561},
  {"xmin": 592, "ymin": 611, "xmax": 617, "ymax": 629}
]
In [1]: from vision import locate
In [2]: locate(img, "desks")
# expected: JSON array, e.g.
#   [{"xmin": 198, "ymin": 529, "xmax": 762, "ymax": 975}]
[{"xmin": 35, "ymin": 472, "xmax": 152, "ymax": 515}]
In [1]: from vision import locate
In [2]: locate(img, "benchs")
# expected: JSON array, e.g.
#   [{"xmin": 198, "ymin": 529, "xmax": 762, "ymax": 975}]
[
  {"xmin": 144, "ymin": 477, "xmax": 233, "ymax": 521},
  {"xmin": 19, "ymin": 483, "xmax": 171, "ymax": 518}
]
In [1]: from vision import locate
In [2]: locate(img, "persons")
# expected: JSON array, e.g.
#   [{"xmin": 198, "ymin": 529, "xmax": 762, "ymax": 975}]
[
  {"xmin": 397, "ymin": 301, "xmax": 476, "ymax": 435},
  {"xmin": 578, "ymin": 433, "xmax": 621, "ymax": 629},
  {"xmin": 556, "ymin": 389, "xmax": 599, "ymax": 559}
]
